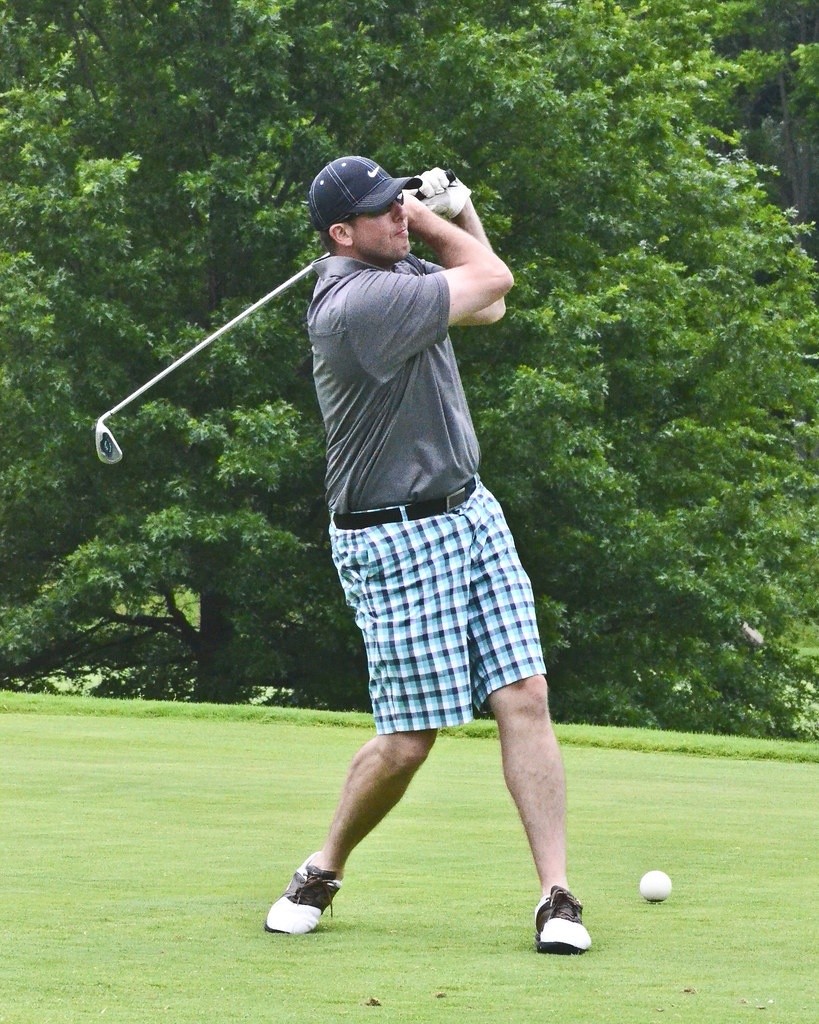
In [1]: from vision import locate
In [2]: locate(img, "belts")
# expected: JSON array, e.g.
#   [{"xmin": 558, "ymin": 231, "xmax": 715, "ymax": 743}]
[{"xmin": 329, "ymin": 478, "xmax": 476, "ymax": 530}]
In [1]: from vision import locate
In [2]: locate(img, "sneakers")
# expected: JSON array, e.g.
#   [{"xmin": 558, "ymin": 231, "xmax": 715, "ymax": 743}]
[
  {"xmin": 265, "ymin": 850, "xmax": 342, "ymax": 935},
  {"xmin": 533, "ymin": 886, "xmax": 591, "ymax": 955}
]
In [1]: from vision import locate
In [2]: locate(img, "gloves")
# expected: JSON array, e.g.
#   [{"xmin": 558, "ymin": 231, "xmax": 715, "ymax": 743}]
[{"xmin": 403, "ymin": 167, "xmax": 472, "ymax": 218}]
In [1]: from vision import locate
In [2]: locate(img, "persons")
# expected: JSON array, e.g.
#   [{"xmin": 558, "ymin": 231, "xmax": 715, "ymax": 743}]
[{"xmin": 265, "ymin": 156, "xmax": 591, "ymax": 955}]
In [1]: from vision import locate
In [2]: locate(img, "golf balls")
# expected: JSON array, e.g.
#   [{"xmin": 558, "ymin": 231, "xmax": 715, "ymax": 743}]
[{"xmin": 640, "ymin": 868, "xmax": 673, "ymax": 902}]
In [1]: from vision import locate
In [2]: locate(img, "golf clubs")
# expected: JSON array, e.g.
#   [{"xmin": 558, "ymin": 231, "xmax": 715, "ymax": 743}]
[{"xmin": 93, "ymin": 166, "xmax": 458, "ymax": 466}]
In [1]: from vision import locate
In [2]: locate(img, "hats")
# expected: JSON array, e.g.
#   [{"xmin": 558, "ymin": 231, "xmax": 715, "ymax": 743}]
[{"xmin": 308, "ymin": 156, "xmax": 422, "ymax": 231}]
